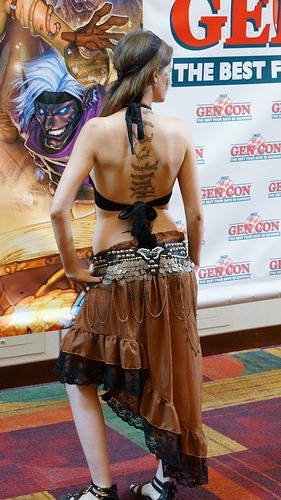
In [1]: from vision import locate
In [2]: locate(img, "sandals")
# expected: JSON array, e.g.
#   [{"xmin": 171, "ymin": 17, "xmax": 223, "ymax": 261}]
[
  {"xmin": 128, "ymin": 475, "xmax": 177, "ymax": 500},
  {"xmin": 59, "ymin": 479, "xmax": 118, "ymax": 500}
]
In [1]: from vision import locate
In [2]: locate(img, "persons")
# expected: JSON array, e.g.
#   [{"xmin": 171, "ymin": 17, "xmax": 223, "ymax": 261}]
[{"xmin": 50, "ymin": 30, "xmax": 210, "ymax": 500}]
